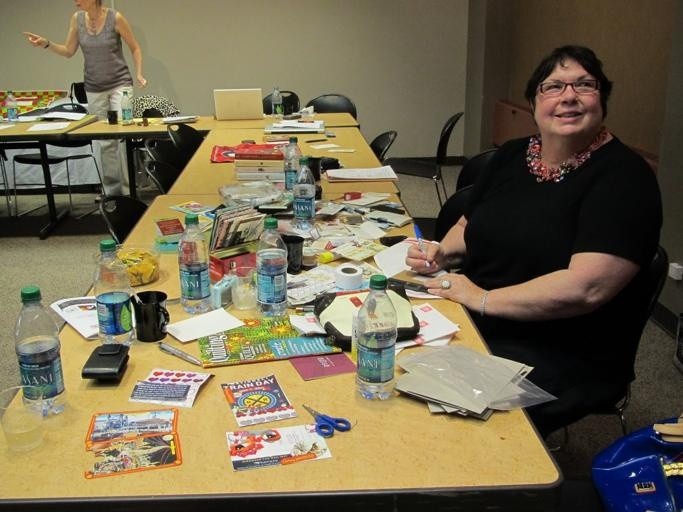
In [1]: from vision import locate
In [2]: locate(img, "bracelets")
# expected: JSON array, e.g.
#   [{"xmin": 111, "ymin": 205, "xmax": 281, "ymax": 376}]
[
  {"xmin": 44, "ymin": 41, "xmax": 49, "ymax": 49},
  {"xmin": 480, "ymin": 290, "xmax": 488, "ymax": 317}
]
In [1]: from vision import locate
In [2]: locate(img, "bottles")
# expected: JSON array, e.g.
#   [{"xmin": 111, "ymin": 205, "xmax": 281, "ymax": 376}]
[
  {"xmin": 256, "ymin": 217, "xmax": 288, "ymax": 315},
  {"xmin": 93, "ymin": 239, "xmax": 135, "ymax": 346},
  {"xmin": 178, "ymin": 214, "xmax": 211, "ymax": 313},
  {"xmin": 292, "ymin": 156, "xmax": 315, "ymax": 231},
  {"xmin": 356, "ymin": 275, "xmax": 398, "ymax": 400},
  {"xmin": 271, "ymin": 86, "xmax": 284, "ymax": 122},
  {"xmin": 5, "ymin": 91, "xmax": 18, "ymax": 125},
  {"xmin": 284, "ymin": 137, "xmax": 301, "ymax": 191},
  {"xmin": 14, "ymin": 285, "xmax": 66, "ymax": 417},
  {"xmin": 121, "ymin": 91, "xmax": 133, "ymax": 123}
]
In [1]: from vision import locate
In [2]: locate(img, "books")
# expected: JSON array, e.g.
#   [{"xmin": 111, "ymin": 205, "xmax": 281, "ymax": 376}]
[
  {"xmin": 199, "ymin": 317, "xmax": 343, "ymax": 368},
  {"xmin": 233, "ymin": 144, "xmax": 285, "ymax": 184},
  {"xmin": 209, "ymin": 205, "xmax": 266, "ymax": 253}
]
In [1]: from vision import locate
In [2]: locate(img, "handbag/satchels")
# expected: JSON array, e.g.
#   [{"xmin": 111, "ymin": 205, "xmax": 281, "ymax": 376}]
[
  {"xmin": 591, "ymin": 415, "xmax": 682, "ymax": 512},
  {"xmin": 70, "ymin": 82, "xmax": 88, "ymax": 112}
]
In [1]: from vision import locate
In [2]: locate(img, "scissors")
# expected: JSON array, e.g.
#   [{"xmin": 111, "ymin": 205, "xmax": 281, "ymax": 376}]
[{"xmin": 302, "ymin": 404, "xmax": 351, "ymax": 438}]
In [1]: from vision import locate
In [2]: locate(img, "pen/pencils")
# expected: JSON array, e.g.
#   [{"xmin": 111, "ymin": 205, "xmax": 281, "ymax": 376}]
[
  {"xmin": 370, "ymin": 218, "xmax": 394, "ymax": 224},
  {"xmin": 306, "ymin": 139, "xmax": 328, "ymax": 142},
  {"xmin": 159, "ymin": 342, "xmax": 201, "ymax": 366},
  {"xmin": 354, "ymin": 209, "xmax": 365, "ymax": 215},
  {"xmin": 298, "ymin": 120, "xmax": 314, "ymax": 123},
  {"xmin": 414, "ymin": 224, "xmax": 431, "ymax": 267}
]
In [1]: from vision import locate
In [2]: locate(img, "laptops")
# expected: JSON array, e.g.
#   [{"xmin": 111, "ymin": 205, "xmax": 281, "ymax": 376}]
[{"xmin": 213, "ymin": 88, "xmax": 265, "ymax": 120}]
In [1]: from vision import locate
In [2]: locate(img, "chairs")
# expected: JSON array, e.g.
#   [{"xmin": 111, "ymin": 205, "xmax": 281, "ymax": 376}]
[{"xmin": 0, "ymin": 82, "xmax": 204, "ymax": 246}]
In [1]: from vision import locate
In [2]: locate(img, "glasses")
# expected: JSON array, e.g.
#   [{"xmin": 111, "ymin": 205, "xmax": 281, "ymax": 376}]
[{"xmin": 536, "ymin": 79, "xmax": 602, "ymax": 98}]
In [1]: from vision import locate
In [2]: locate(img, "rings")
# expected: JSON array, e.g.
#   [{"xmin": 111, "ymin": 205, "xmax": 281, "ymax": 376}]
[{"xmin": 442, "ymin": 280, "xmax": 451, "ymax": 289}]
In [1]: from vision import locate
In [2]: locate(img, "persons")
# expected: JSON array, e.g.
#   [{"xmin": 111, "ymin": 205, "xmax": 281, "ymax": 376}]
[
  {"xmin": 23, "ymin": 0, "xmax": 147, "ymax": 202},
  {"xmin": 405, "ymin": 45, "xmax": 663, "ymax": 436}
]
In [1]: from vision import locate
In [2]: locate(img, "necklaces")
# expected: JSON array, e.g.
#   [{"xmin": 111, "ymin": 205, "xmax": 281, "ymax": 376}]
[{"xmin": 525, "ymin": 126, "xmax": 608, "ymax": 184}]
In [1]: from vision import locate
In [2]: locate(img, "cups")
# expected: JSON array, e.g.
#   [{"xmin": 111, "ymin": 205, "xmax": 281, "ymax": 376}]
[
  {"xmin": 230, "ymin": 266, "xmax": 256, "ymax": 312},
  {"xmin": 107, "ymin": 110, "xmax": 118, "ymax": 124},
  {"xmin": 283, "ymin": 236, "xmax": 305, "ymax": 274},
  {"xmin": 131, "ymin": 291, "xmax": 169, "ymax": 342},
  {"xmin": 309, "ymin": 158, "xmax": 321, "ymax": 181},
  {"xmin": 0, "ymin": 385, "xmax": 44, "ymax": 452}
]
